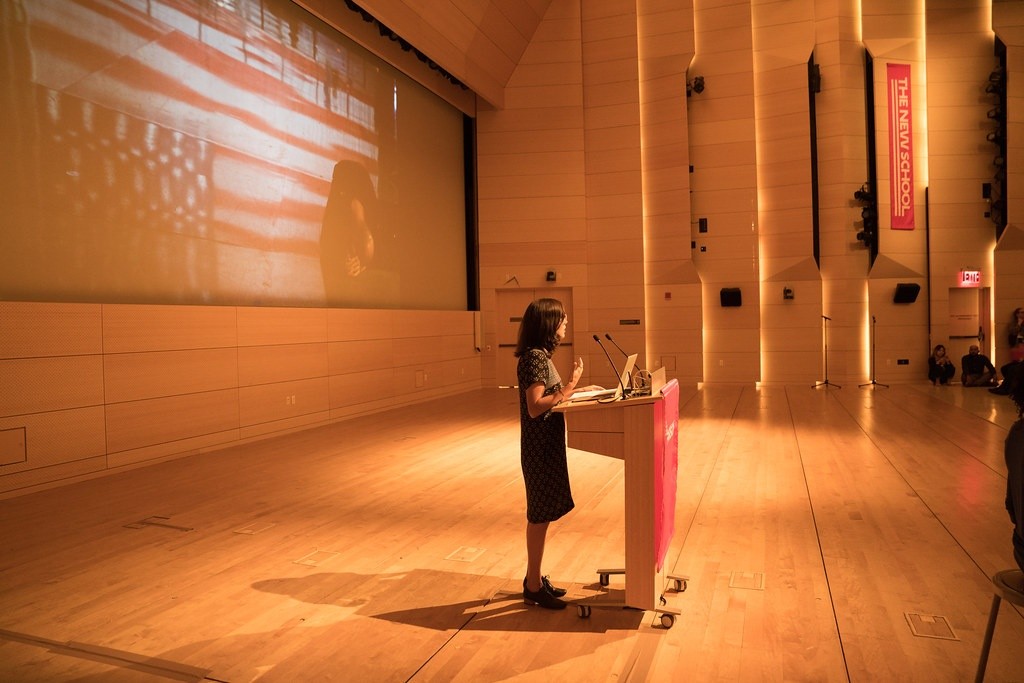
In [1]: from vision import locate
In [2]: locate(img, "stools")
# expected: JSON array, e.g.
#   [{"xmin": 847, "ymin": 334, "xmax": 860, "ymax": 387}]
[{"xmin": 973, "ymin": 567, "xmax": 1024, "ymax": 682}]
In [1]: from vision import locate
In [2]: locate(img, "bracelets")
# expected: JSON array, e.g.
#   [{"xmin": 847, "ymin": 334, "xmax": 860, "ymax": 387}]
[{"xmin": 558, "ymin": 389, "xmax": 565, "ymax": 398}]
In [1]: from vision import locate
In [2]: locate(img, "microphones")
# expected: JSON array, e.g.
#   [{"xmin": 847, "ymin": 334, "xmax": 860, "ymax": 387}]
[
  {"xmin": 593, "ymin": 335, "xmax": 627, "ymax": 400},
  {"xmin": 605, "ymin": 333, "xmax": 646, "ymax": 388},
  {"xmin": 821, "ymin": 315, "xmax": 832, "ymax": 320},
  {"xmin": 872, "ymin": 314, "xmax": 876, "ymax": 323}
]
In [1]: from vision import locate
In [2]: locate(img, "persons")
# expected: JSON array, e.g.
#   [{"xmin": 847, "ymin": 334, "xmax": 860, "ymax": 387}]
[
  {"xmin": 928, "ymin": 344, "xmax": 956, "ymax": 388},
  {"xmin": 512, "ymin": 298, "xmax": 583, "ymax": 609},
  {"xmin": 1007, "ymin": 308, "xmax": 1024, "ymax": 363},
  {"xmin": 960, "ymin": 345, "xmax": 1000, "ymax": 387},
  {"xmin": 1003, "ymin": 363, "xmax": 1024, "ymax": 572}
]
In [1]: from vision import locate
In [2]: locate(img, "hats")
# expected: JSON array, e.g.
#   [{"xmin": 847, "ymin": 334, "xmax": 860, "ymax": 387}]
[{"xmin": 988, "ymin": 362, "xmax": 1024, "ymax": 396}]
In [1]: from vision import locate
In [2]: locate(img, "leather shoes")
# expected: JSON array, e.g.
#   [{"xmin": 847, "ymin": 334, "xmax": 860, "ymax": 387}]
[{"xmin": 523, "ymin": 577, "xmax": 567, "ymax": 609}]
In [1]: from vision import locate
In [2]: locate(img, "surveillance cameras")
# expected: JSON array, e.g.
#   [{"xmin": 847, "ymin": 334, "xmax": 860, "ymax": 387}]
[{"xmin": 693, "ymin": 77, "xmax": 705, "ymax": 94}]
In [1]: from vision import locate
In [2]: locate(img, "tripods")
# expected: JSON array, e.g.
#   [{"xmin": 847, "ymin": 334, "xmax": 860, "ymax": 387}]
[
  {"xmin": 811, "ymin": 319, "xmax": 841, "ymax": 389},
  {"xmin": 858, "ymin": 322, "xmax": 889, "ymax": 390}
]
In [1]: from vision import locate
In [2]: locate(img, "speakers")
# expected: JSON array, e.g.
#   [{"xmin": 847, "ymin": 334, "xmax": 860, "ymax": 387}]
[
  {"xmin": 894, "ymin": 283, "xmax": 920, "ymax": 303},
  {"xmin": 720, "ymin": 288, "xmax": 742, "ymax": 307}
]
